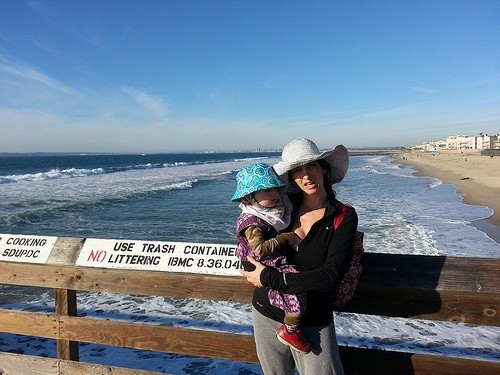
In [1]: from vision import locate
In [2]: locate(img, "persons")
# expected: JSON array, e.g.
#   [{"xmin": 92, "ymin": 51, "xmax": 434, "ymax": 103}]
[
  {"xmin": 231, "ymin": 163, "xmax": 311, "ymax": 354},
  {"xmin": 239, "ymin": 138, "xmax": 359, "ymax": 375}
]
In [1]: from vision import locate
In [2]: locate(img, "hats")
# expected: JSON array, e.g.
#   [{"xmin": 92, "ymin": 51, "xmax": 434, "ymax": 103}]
[
  {"xmin": 231, "ymin": 163, "xmax": 286, "ymax": 202},
  {"xmin": 271, "ymin": 138, "xmax": 349, "ymax": 192}
]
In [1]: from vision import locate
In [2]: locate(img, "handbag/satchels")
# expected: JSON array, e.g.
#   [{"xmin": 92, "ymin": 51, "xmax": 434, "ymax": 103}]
[{"xmin": 330, "ymin": 205, "xmax": 364, "ymax": 310}]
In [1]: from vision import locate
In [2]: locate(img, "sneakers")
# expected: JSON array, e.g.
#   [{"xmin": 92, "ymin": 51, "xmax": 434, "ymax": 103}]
[{"xmin": 276, "ymin": 325, "xmax": 311, "ymax": 354}]
